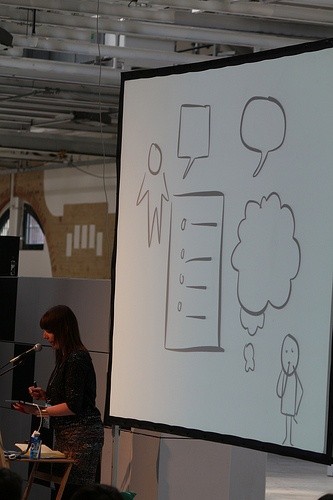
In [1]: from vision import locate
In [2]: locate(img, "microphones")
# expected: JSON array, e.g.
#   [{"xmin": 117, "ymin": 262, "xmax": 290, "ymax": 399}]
[{"xmin": 11, "ymin": 343, "xmax": 42, "ymax": 363}]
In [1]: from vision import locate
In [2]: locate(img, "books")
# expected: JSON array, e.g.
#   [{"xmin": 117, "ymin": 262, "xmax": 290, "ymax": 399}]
[{"xmin": 14, "ymin": 443, "xmax": 66, "ymax": 459}]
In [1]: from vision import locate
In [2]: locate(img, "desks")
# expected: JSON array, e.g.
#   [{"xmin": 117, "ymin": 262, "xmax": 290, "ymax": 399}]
[{"xmin": 17, "ymin": 458, "xmax": 80, "ymax": 500}]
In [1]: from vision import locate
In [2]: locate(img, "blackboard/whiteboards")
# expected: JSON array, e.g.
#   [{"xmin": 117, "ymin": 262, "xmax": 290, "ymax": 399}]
[{"xmin": 101, "ymin": 37, "xmax": 333, "ymax": 467}]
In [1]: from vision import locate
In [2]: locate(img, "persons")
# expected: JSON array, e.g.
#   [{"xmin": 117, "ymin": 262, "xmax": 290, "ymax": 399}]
[
  {"xmin": 0, "ymin": 431, "xmax": 23, "ymax": 500},
  {"xmin": 71, "ymin": 484, "xmax": 125, "ymax": 500},
  {"xmin": 11, "ymin": 305, "xmax": 104, "ymax": 500}
]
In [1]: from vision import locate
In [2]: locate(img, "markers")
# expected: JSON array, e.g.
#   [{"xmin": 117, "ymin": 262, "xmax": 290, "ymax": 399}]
[{"xmin": 34, "ymin": 381, "xmax": 39, "ymax": 401}]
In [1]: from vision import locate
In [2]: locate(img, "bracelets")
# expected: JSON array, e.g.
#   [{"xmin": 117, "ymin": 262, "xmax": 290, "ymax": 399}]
[{"xmin": 36, "ymin": 408, "xmax": 49, "ymax": 418}]
